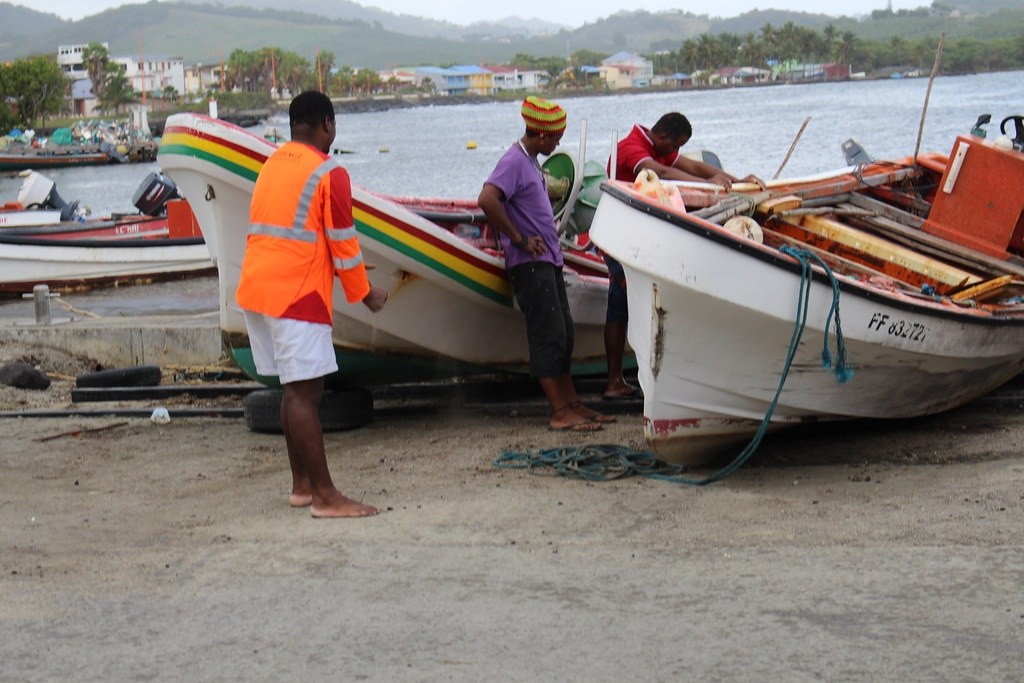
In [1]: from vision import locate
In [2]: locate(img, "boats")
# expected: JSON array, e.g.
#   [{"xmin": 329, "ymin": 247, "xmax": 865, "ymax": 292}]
[
  {"xmin": 588, "ymin": 111, "xmax": 1024, "ymax": 470},
  {"xmin": 8, "ymin": 213, "xmax": 169, "ymax": 241},
  {"xmin": 158, "ymin": 112, "xmax": 725, "ymax": 392},
  {"xmin": 0, "ymin": 147, "xmax": 150, "ymax": 170},
  {"xmin": 0, "ymin": 233, "xmax": 218, "ymax": 293},
  {"xmin": 0, "ymin": 209, "xmax": 62, "ymax": 227}
]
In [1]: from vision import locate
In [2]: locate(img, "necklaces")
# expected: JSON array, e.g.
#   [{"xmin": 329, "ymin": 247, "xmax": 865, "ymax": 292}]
[{"xmin": 517, "ymin": 140, "xmax": 543, "ymax": 180}]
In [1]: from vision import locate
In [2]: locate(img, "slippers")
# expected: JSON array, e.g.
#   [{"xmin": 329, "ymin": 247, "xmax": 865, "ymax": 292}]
[
  {"xmin": 584, "ymin": 410, "xmax": 618, "ymax": 423},
  {"xmin": 547, "ymin": 416, "xmax": 605, "ymax": 431},
  {"xmin": 602, "ymin": 384, "xmax": 644, "ymax": 400}
]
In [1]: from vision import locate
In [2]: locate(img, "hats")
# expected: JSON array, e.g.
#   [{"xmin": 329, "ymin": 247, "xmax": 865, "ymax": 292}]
[{"xmin": 520, "ymin": 95, "xmax": 566, "ymax": 134}]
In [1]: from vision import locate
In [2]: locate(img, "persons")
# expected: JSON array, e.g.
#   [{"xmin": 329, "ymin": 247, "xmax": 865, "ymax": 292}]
[
  {"xmin": 234, "ymin": 91, "xmax": 387, "ymax": 517},
  {"xmin": 477, "ymin": 94, "xmax": 618, "ymax": 432},
  {"xmin": 604, "ymin": 112, "xmax": 767, "ymax": 398}
]
